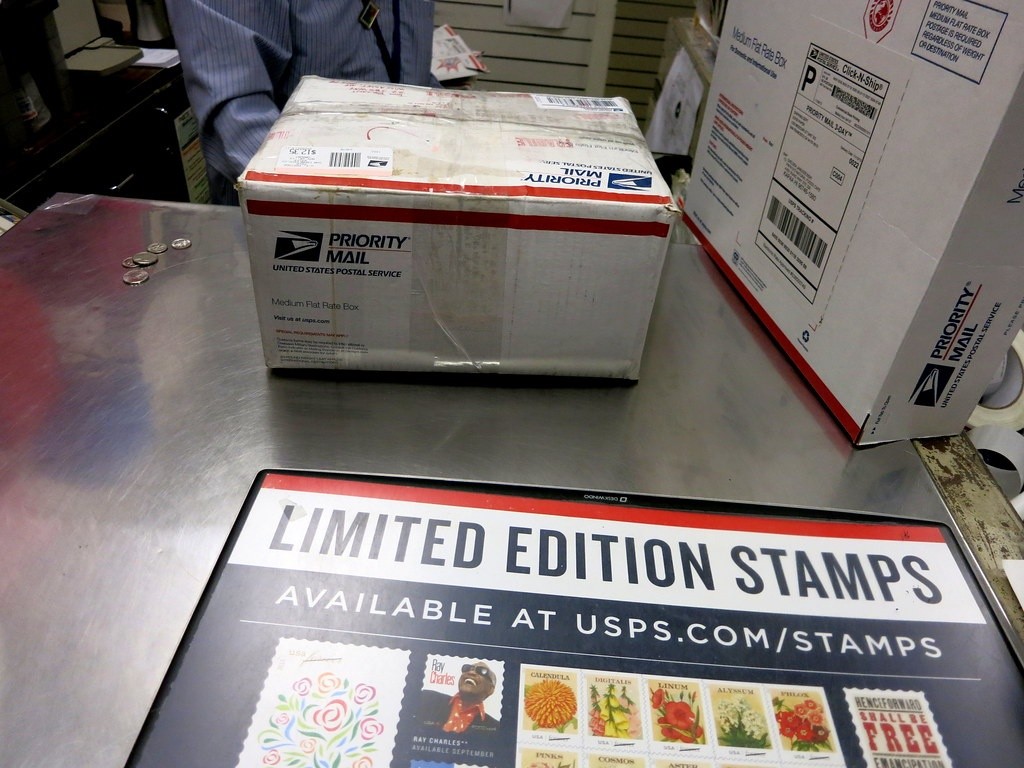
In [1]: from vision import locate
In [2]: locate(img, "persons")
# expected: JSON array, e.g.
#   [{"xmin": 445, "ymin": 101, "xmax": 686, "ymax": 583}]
[
  {"xmin": 419, "ymin": 662, "xmax": 499, "ymax": 733},
  {"xmin": 165, "ymin": 0, "xmax": 446, "ymax": 206}
]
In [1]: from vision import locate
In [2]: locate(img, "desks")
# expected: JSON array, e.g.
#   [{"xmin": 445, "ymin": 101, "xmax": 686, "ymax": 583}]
[{"xmin": 0, "ymin": 192, "xmax": 1024, "ymax": 768}]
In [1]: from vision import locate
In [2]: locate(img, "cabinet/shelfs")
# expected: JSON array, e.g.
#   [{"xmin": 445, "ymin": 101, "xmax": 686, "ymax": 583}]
[{"xmin": 88, "ymin": 86, "xmax": 213, "ymax": 206}]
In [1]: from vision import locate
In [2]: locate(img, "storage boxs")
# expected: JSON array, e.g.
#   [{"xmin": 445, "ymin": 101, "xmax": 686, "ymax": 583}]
[
  {"xmin": 676, "ymin": 0, "xmax": 1024, "ymax": 449},
  {"xmin": 235, "ymin": 76, "xmax": 682, "ymax": 382}
]
